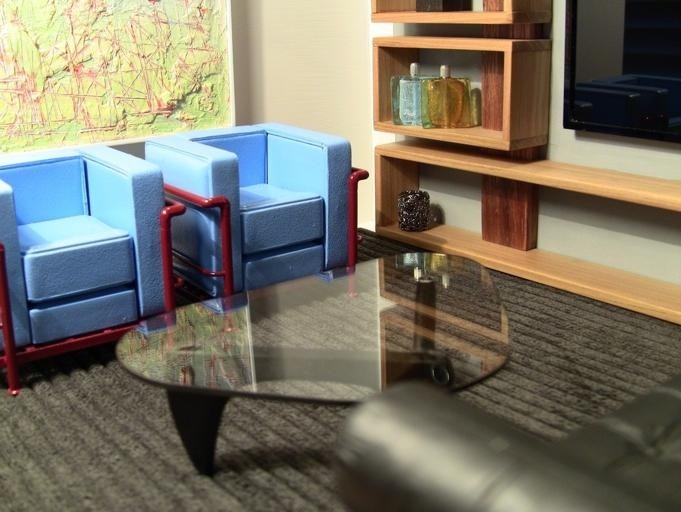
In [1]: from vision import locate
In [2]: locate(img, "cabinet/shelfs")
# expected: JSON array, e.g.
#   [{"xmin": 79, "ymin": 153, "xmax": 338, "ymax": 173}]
[
  {"xmin": 376, "ymin": 140, "xmax": 680, "ymax": 324},
  {"xmin": 372, "ymin": 1, "xmax": 553, "ymax": 25},
  {"xmin": 373, "ymin": 37, "xmax": 551, "ymax": 150}
]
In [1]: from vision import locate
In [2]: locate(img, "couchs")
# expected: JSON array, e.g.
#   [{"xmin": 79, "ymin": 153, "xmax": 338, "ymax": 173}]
[
  {"xmin": 144, "ymin": 123, "xmax": 370, "ymax": 331},
  {"xmin": 2, "ymin": 145, "xmax": 187, "ymax": 398}
]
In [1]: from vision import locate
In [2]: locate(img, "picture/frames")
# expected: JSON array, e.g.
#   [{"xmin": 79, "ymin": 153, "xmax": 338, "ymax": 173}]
[{"xmin": 0, "ymin": 2, "xmax": 236, "ymax": 171}]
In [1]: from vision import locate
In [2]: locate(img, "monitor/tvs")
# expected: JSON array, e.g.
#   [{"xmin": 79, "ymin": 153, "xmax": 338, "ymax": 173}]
[{"xmin": 563, "ymin": 0, "xmax": 681, "ymax": 144}]
